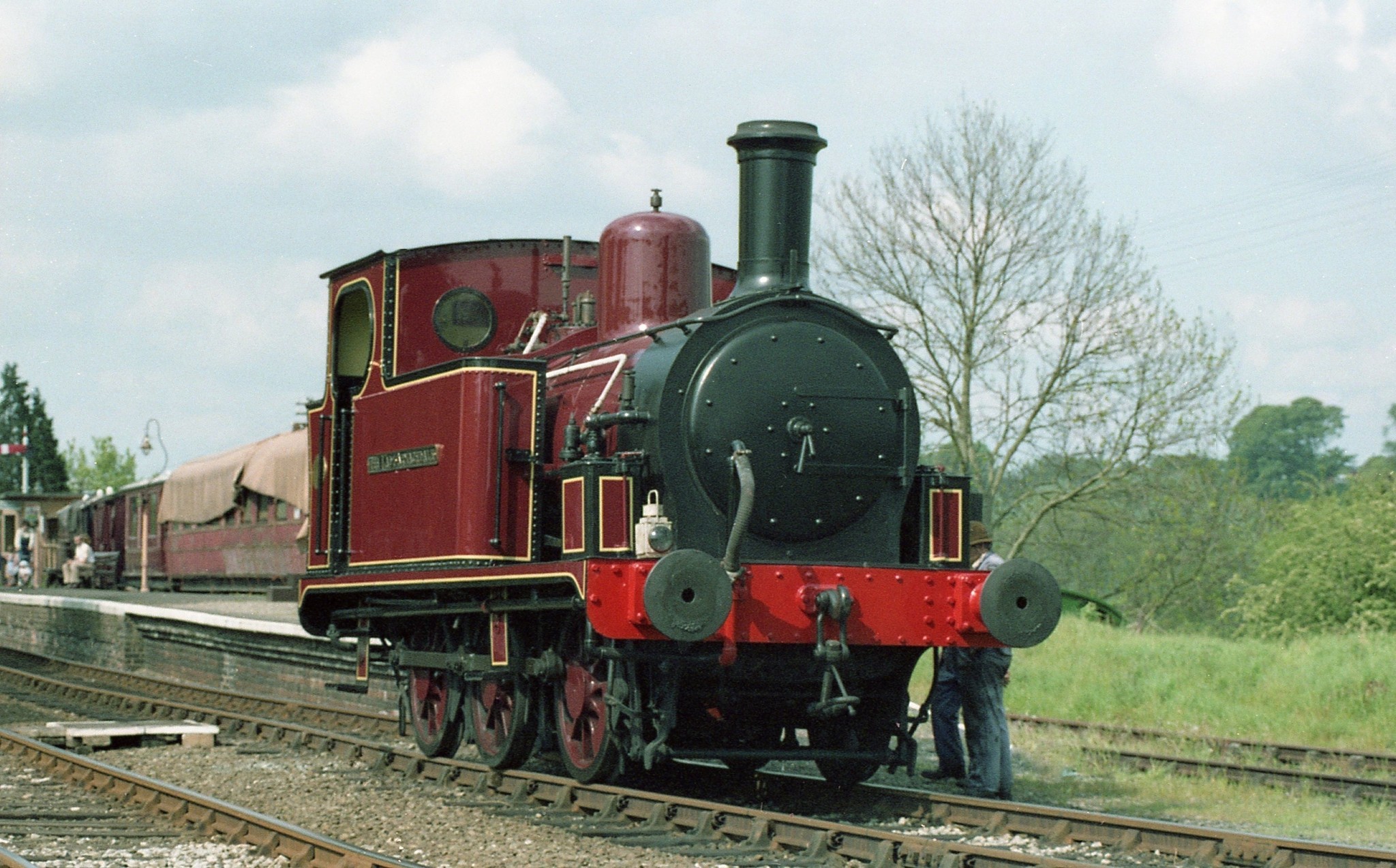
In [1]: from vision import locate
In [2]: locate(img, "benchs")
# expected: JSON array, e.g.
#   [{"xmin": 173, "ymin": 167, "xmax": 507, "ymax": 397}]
[{"xmin": 42, "ymin": 552, "xmax": 119, "ymax": 591}]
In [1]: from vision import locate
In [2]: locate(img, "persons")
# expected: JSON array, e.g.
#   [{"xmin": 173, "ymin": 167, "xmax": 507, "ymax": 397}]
[
  {"xmin": 6, "ymin": 519, "xmax": 38, "ymax": 591},
  {"xmin": 61, "ymin": 535, "xmax": 96, "ymax": 591},
  {"xmin": 921, "ymin": 521, "xmax": 1015, "ymax": 801}
]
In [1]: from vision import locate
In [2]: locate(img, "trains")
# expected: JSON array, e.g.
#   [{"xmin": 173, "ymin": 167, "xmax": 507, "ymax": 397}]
[{"xmin": 88, "ymin": 117, "xmax": 1064, "ymax": 790}]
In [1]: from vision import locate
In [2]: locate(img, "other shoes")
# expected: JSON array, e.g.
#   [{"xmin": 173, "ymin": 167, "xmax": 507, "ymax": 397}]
[
  {"xmin": 65, "ymin": 583, "xmax": 81, "ymax": 588},
  {"xmin": 951, "ymin": 779, "xmax": 1013, "ymax": 800},
  {"xmin": 921, "ymin": 764, "xmax": 966, "ymax": 781}
]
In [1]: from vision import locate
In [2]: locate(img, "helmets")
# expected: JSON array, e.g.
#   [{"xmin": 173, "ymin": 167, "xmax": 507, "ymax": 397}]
[{"xmin": 969, "ymin": 521, "xmax": 991, "ymax": 546}]
